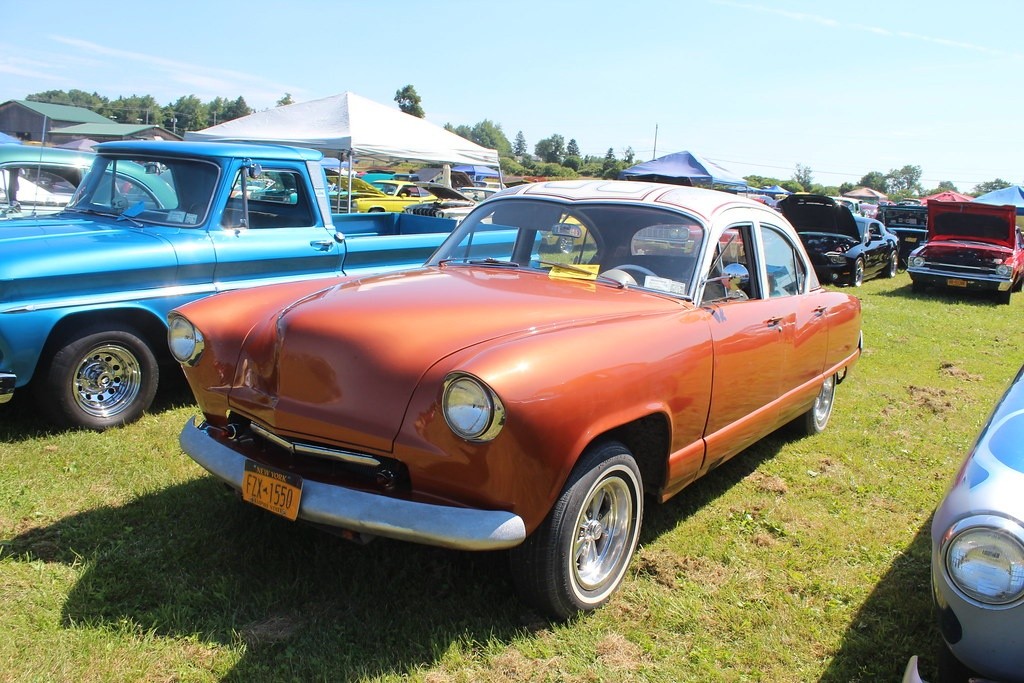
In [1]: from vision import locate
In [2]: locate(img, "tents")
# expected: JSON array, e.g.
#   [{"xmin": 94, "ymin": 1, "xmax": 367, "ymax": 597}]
[
  {"xmin": 729, "ymin": 182, "xmax": 793, "ymax": 197},
  {"xmin": 450, "ymin": 164, "xmax": 500, "ymax": 182},
  {"xmin": 971, "ymin": 185, "xmax": 1024, "ymax": 216},
  {"xmin": 616, "ymin": 149, "xmax": 748, "ymax": 198},
  {"xmin": 842, "ymin": 187, "xmax": 889, "ymax": 201},
  {"xmin": 183, "ymin": 91, "xmax": 503, "ymax": 214},
  {"xmin": 46, "ymin": 122, "xmax": 185, "ymax": 141},
  {"xmin": 0, "ymin": 99, "xmax": 118, "ymax": 142},
  {"xmin": 920, "ymin": 189, "xmax": 974, "ymax": 205}
]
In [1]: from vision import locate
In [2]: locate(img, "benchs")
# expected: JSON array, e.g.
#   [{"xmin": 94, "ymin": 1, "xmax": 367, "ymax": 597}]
[
  {"xmin": 190, "ymin": 203, "xmax": 281, "ymax": 227},
  {"xmin": 596, "ymin": 254, "xmax": 728, "ymax": 301}
]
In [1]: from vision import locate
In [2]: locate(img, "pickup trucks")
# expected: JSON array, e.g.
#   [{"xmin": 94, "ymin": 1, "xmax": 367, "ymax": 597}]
[{"xmin": 0, "ymin": 140, "xmax": 543, "ymax": 436}]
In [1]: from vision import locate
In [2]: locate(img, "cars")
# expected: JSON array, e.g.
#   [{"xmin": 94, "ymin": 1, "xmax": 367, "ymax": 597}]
[
  {"xmin": 164, "ymin": 179, "xmax": 868, "ymax": 624},
  {"xmin": 775, "ymin": 192, "xmax": 900, "ymax": 288},
  {"xmin": 232, "ymin": 169, "xmax": 511, "ymax": 229},
  {"xmin": 541, "ymin": 210, "xmax": 600, "ymax": 254},
  {"xmin": 878, "ymin": 204, "xmax": 929, "ymax": 260},
  {"xmin": 0, "ymin": 145, "xmax": 178, "ymax": 224},
  {"xmin": 906, "ymin": 197, "xmax": 1024, "ymax": 303},
  {"xmin": 632, "ymin": 197, "xmax": 770, "ymax": 255},
  {"xmin": 930, "ymin": 362, "xmax": 1023, "ymax": 683}
]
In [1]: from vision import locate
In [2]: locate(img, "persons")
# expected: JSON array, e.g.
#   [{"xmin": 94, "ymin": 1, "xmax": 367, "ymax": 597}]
[{"xmin": 1015, "ymin": 227, "xmax": 1023, "ymax": 250}]
